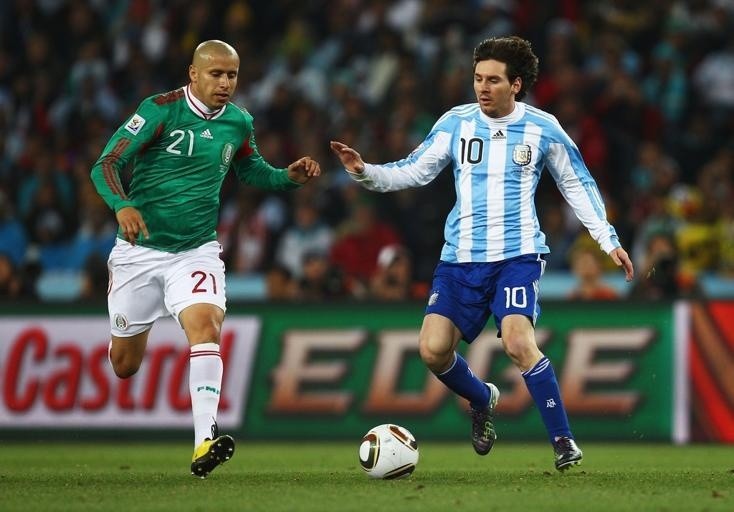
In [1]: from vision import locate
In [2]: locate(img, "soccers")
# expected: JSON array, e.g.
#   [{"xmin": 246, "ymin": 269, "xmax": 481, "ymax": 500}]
[{"xmin": 359, "ymin": 423, "xmax": 419, "ymax": 479}]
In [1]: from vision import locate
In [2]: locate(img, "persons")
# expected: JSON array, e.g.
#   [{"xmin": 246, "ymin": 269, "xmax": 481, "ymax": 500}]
[
  {"xmin": 89, "ymin": 40, "xmax": 321, "ymax": 480},
  {"xmin": 2, "ymin": 1, "xmax": 734, "ymax": 302},
  {"xmin": 330, "ymin": 34, "xmax": 635, "ymax": 474}
]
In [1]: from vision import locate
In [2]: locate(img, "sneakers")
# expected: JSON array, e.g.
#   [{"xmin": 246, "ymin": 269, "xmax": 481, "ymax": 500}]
[
  {"xmin": 553, "ymin": 434, "xmax": 584, "ymax": 472},
  {"xmin": 191, "ymin": 417, "xmax": 236, "ymax": 480},
  {"xmin": 466, "ymin": 382, "xmax": 502, "ymax": 457}
]
